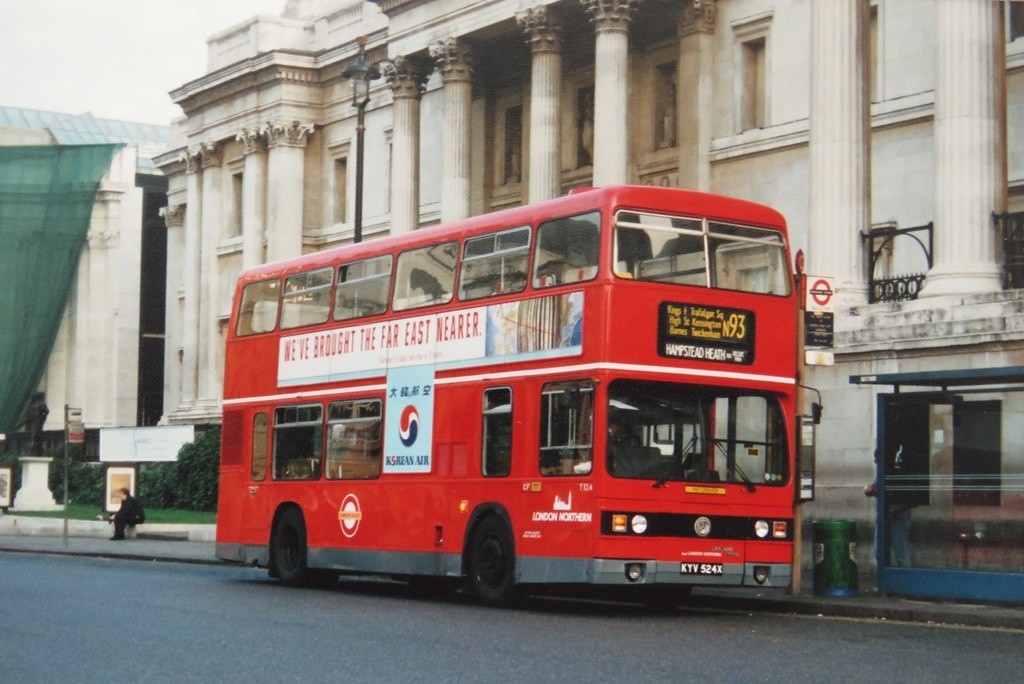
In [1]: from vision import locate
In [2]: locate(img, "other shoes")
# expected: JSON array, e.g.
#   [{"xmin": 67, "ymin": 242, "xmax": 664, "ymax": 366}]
[{"xmin": 109, "ymin": 536, "xmax": 125, "ymax": 540}]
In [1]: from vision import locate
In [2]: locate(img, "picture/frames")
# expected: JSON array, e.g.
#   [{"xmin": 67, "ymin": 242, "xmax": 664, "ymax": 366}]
[{"xmin": 102, "ymin": 462, "xmax": 138, "ymax": 514}]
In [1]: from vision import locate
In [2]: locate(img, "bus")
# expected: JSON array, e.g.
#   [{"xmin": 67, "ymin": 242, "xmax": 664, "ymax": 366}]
[{"xmin": 207, "ymin": 183, "xmax": 828, "ymax": 608}]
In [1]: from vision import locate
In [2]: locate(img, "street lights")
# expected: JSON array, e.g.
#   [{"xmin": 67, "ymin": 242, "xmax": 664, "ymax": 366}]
[{"xmin": 341, "ymin": 34, "xmax": 382, "ymax": 244}]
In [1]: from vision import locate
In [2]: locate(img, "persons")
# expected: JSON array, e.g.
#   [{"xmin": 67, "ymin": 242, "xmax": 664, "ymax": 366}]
[
  {"xmin": 108, "ymin": 488, "xmax": 145, "ymax": 541},
  {"xmin": 865, "ymin": 449, "xmax": 910, "ymax": 594}
]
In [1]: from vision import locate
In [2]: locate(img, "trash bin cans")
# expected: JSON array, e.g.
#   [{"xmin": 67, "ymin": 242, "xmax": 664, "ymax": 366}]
[{"xmin": 811, "ymin": 519, "xmax": 859, "ymax": 595}]
